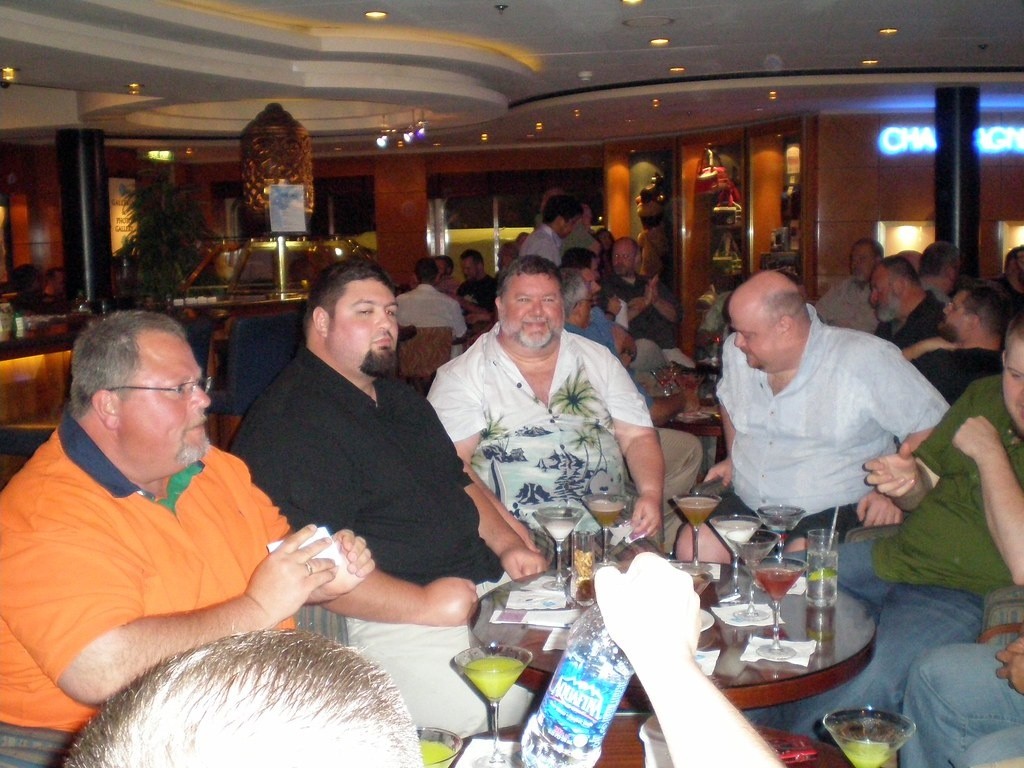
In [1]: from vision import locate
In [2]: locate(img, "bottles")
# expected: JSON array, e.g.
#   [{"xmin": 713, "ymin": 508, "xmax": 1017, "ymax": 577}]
[
  {"xmin": 13, "ymin": 312, "xmax": 26, "ymax": 341},
  {"xmin": 614, "ymin": 297, "xmax": 629, "ymax": 332},
  {"xmin": 521, "ymin": 602, "xmax": 635, "ymax": 768}
]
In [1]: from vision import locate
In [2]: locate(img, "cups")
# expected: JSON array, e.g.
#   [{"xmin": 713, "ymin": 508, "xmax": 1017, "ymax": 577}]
[
  {"xmin": 572, "ymin": 532, "xmax": 596, "ymax": 606},
  {"xmin": 823, "ymin": 706, "xmax": 916, "ymax": 768},
  {"xmin": 415, "ymin": 726, "xmax": 464, "ymax": 768},
  {"xmin": 806, "ymin": 527, "xmax": 840, "ymax": 608}
]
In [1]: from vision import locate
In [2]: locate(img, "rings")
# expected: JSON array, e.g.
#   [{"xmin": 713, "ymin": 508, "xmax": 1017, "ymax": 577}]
[{"xmin": 306, "ymin": 563, "xmax": 312, "ymax": 575}]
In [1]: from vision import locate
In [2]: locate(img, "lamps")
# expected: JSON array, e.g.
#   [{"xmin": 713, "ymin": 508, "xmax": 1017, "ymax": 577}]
[
  {"xmin": 376, "ymin": 113, "xmax": 388, "ymax": 148},
  {"xmin": 403, "ymin": 108, "xmax": 427, "ymax": 142}
]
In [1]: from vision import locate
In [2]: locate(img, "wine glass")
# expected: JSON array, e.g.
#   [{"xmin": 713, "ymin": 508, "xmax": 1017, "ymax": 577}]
[
  {"xmin": 672, "ymin": 494, "xmax": 722, "ymax": 574},
  {"xmin": 454, "ymin": 645, "xmax": 533, "ymax": 768},
  {"xmin": 755, "ymin": 505, "xmax": 810, "ymax": 588},
  {"xmin": 652, "ymin": 365, "xmax": 677, "ymax": 395},
  {"xmin": 535, "ymin": 506, "xmax": 585, "ymax": 590},
  {"xmin": 709, "ymin": 515, "xmax": 810, "ymax": 660},
  {"xmin": 582, "ymin": 493, "xmax": 630, "ymax": 571}
]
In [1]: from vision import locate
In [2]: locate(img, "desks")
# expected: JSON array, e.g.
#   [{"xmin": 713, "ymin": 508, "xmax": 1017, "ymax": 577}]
[
  {"xmin": 657, "ymin": 398, "xmax": 726, "ymax": 483},
  {"xmin": 448, "ymin": 716, "xmax": 885, "ymax": 768},
  {"xmin": 468, "ymin": 562, "xmax": 876, "ymax": 711}
]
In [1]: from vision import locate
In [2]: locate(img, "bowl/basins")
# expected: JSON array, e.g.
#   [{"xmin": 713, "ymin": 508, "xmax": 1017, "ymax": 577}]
[{"xmin": 0, "ymin": 319, "xmax": 14, "ymax": 342}]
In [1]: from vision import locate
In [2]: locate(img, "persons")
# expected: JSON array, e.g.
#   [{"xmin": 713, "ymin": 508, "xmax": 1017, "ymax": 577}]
[
  {"xmin": 62, "ymin": 551, "xmax": 790, "ymax": 767},
  {"xmin": 995, "ymin": 245, "xmax": 1024, "ymax": 315},
  {"xmin": 919, "ymin": 241, "xmax": 961, "ymax": 304},
  {"xmin": 747, "ymin": 311, "xmax": 1023, "ymax": 767},
  {"xmin": 225, "ymin": 262, "xmax": 549, "ymax": 739},
  {"xmin": 695, "ymin": 290, "xmax": 735, "ymax": 363},
  {"xmin": 0, "ymin": 312, "xmax": 376, "ymax": 768},
  {"xmin": 519, "ymin": 188, "xmax": 701, "ymax": 554},
  {"xmin": 814, "ymin": 237, "xmax": 883, "ymax": 334},
  {"xmin": 427, "ymin": 254, "xmax": 665, "ymax": 561},
  {"xmin": 902, "ymin": 279, "xmax": 1012, "ymax": 406},
  {"xmin": 676, "ymin": 270, "xmax": 951, "ymax": 564},
  {"xmin": 395, "ymin": 258, "xmax": 467, "ymax": 341},
  {"xmin": 12, "ymin": 265, "xmax": 67, "ymax": 314},
  {"xmin": 873, "ymin": 256, "xmax": 946, "ymax": 378},
  {"xmin": 435, "ymin": 231, "xmax": 528, "ymax": 342}
]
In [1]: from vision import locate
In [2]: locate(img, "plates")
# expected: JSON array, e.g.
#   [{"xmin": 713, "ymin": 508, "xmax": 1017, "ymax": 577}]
[{"xmin": 699, "ymin": 609, "xmax": 715, "ymax": 634}]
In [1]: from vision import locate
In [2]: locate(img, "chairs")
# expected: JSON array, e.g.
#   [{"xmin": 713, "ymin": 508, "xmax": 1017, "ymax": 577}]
[
  {"xmin": 188, "ymin": 314, "xmax": 302, "ymax": 451},
  {"xmin": 398, "ymin": 326, "xmax": 453, "ymax": 396}
]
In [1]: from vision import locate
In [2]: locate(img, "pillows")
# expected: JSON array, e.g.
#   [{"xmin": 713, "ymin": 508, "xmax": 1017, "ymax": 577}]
[{"xmin": 482, "ymin": 421, "xmax": 625, "ymax": 530}]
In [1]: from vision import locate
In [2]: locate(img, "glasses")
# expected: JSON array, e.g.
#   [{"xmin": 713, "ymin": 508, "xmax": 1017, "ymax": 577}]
[{"xmin": 108, "ymin": 377, "xmax": 211, "ymax": 400}]
